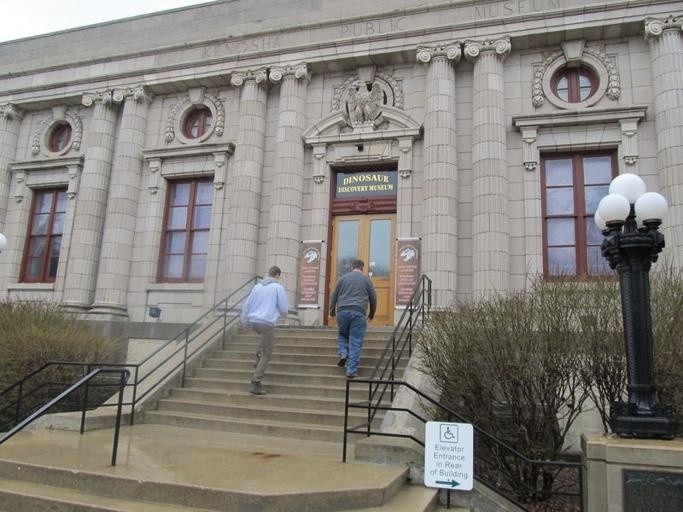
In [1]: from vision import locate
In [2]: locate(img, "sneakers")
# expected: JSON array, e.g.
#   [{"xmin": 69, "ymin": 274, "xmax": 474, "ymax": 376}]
[
  {"xmin": 337, "ymin": 353, "xmax": 348, "ymax": 366},
  {"xmin": 249, "ymin": 380, "xmax": 266, "ymax": 395},
  {"xmin": 253, "ymin": 353, "xmax": 261, "ymax": 367},
  {"xmin": 344, "ymin": 370, "xmax": 359, "ymax": 379}
]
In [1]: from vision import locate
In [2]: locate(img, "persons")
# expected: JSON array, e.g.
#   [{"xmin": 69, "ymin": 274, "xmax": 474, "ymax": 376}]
[
  {"xmin": 240, "ymin": 265, "xmax": 289, "ymax": 395},
  {"xmin": 327, "ymin": 259, "xmax": 378, "ymax": 377}
]
